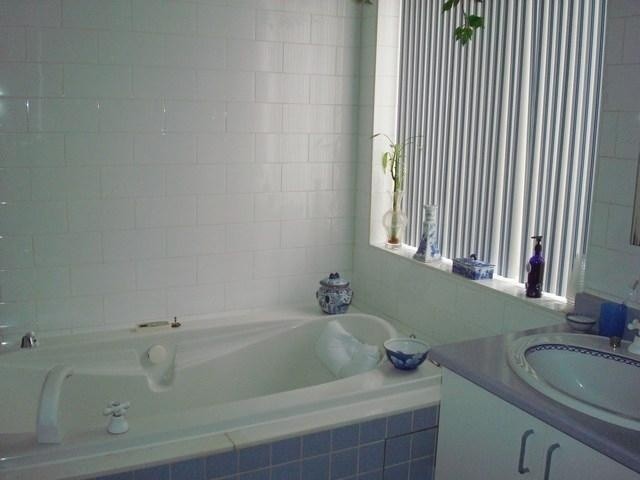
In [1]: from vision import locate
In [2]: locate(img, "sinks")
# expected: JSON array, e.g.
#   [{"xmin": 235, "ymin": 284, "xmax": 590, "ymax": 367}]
[{"xmin": 507, "ymin": 333, "xmax": 640, "ymax": 432}]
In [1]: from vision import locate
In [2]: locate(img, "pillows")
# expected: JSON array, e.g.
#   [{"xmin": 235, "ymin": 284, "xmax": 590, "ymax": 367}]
[{"xmin": 312, "ymin": 319, "xmax": 381, "ymax": 380}]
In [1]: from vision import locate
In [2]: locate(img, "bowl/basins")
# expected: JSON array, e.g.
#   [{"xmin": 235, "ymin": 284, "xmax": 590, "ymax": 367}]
[
  {"xmin": 382, "ymin": 338, "xmax": 429, "ymax": 371},
  {"xmin": 566, "ymin": 314, "xmax": 597, "ymax": 333}
]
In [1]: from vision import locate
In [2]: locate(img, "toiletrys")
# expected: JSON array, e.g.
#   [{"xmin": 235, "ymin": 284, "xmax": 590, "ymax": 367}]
[{"xmin": 524, "ymin": 234, "xmax": 545, "ymax": 297}]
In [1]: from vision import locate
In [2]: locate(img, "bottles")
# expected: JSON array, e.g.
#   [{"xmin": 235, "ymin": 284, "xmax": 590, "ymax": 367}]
[{"xmin": 316, "ymin": 272, "xmax": 353, "ymax": 315}]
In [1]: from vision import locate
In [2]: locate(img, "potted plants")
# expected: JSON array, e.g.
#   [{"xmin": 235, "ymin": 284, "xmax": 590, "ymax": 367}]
[{"xmin": 369, "ymin": 126, "xmax": 424, "ymax": 248}]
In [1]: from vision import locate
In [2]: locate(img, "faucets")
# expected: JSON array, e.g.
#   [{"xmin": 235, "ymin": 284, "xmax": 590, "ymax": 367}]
[
  {"xmin": 21, "ymin": 332, "xmax": 39, "ymax": 348},
  {"xmin": 627, "ymin": 319, "xmax": 640, "ymax": 353},
  {"xmin": 37, "ymin": 364, "xmax": 74, "ymax": 444}
]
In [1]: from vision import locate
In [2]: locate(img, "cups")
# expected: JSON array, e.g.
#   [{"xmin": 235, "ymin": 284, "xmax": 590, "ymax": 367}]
[{"xmin": 599, "ymin": 303, "xmax": 627, "ymax": 337}]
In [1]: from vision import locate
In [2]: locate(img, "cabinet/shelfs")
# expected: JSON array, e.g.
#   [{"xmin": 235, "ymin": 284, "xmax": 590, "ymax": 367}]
[{"xmin": 435, "ymin": 364, "xmax": 640, "ymax": 479}]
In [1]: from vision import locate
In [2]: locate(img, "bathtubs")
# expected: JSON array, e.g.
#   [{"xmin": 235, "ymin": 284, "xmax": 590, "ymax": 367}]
[{"xmin": 0, "ymin": 306, "xmax": 443, "ymax": 480}]
